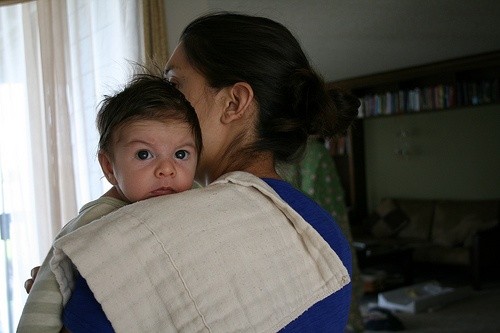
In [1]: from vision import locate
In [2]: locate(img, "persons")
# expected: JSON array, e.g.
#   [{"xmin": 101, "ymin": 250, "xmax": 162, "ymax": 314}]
[
  {"xmin": 25, "ymin": 9, "xmax": 362, "ymax": 333},
  {"xmin": 17, "ymin": 56, "xmax": 204, "ymax": 333}
]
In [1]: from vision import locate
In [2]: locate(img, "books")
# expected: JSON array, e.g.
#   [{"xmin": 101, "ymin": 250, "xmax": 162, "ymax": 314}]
[{"xmin": 358, "ymin": 76, "xmax": 495, "ymax": 116}]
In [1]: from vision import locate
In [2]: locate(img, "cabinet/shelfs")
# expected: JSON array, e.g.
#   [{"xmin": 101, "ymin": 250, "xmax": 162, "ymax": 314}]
[{"xmin": 315, "ymin": 46, "xmax": 499, "ymax": 293}]
